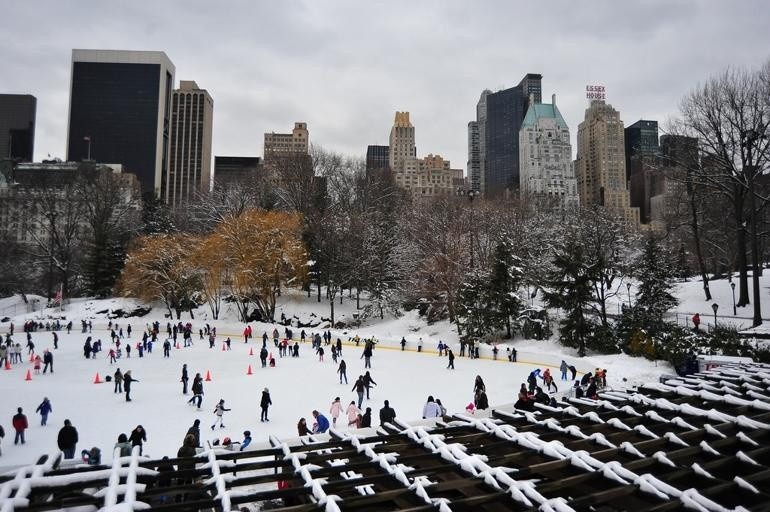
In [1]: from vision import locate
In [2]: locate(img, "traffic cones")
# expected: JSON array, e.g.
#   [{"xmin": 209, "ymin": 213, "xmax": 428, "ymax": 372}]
[
  {"xmin": 203, "ymin": 369, "xmax": 211, "ymax": 382},
  {"xmin": 3, "ymin": 360, "xmax": 11, "ymax": 370},
  {"xmin": 28, "ymin": 352, "xmax": 36, "ymax": 363},
  {"xmin": 93, "ymin": 372, "xmax": 102, "ymax": 384},
  {"xmin": 245, "ymin": 364, "xmax": 254, "ymax": 375},
  {"xmin": 175, "ymin": 342, "xmax": 180, "ymax": 349},
  {"xmin": 221, "ymin": 343, "xmax": 226, "ymax": 352},
  {"xmin": 249, "ymin": 347, "xmax": 254, "ymax": 356},
  {"xmin": 23, "ymin": 369, "xmax": 33, "ymax": 381},
  {"xmin": 267, "ymin": 352, "xmax": 273, "ymax": 361}
]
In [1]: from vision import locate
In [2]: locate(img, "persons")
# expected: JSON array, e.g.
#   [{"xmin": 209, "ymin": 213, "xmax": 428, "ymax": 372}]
[{"xmin": 692, "ymin": 313, "xmax": 701, "ymax": 330}]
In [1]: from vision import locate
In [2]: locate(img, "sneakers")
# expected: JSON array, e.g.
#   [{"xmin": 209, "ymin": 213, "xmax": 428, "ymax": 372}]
[
  {"xmin": 260, "ymin": 418, "xmax": 269, "ymax": 422},
  {"xmin": 211, "ymin": 423, "xmax": 225, "ymax": 431}
]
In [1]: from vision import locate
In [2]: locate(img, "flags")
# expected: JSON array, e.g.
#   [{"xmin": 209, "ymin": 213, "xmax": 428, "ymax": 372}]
[{"xmin": 53, "ymin": 286, "xmax": 61, "ymax": 305}]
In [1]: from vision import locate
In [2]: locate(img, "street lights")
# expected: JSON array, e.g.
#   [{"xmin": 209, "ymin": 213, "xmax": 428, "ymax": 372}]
[
  {"xmin": 625, "ymin": 282, "xmax": 632, "ymax": 310},
  {"xmin": 730, "ymin": 282, "xmax": 740, "ymax": 316},
  {"xmin": 453, "ymin": 188, "xmax": 482, "ymax": 273},
  {"xmin": 711, "ymin": 301, "xmax": 721, "ymax": 331}
]
